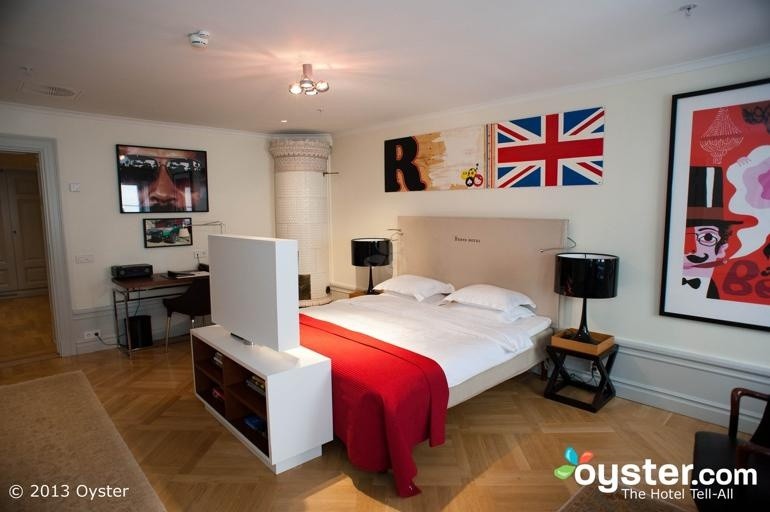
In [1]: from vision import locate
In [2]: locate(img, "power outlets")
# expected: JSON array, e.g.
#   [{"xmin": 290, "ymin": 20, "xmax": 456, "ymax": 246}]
[
  {"xmin": 194, "ymin": 249, "xmax": 202, "ymax": 259},
  {"xmin": 83, "ymin": 330, "xmax": 102, "ymax": 342}
]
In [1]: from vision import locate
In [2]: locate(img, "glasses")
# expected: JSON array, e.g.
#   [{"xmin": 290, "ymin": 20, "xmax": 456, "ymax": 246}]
[
  {"xmin": 118, "ymin": 154, "xmax": 205, "ymax": 189},
  {"xmin": 686, "ymin": 232, "xmax": 719, "ymax": 247}
]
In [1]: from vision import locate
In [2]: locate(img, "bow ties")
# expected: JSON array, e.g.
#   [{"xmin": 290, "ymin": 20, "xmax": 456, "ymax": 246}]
[{"xmin": 682, "ymin": 278, "xmax": 701, "ymax": 289}]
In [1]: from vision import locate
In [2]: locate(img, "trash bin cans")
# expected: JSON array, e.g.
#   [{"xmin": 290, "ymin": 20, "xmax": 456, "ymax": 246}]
[{"xmin": 124, "ymin": 315, "xmax": 153, "ymax": 350}]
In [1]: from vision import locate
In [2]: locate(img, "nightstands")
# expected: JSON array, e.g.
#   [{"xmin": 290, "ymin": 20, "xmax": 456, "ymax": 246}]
[{"xmin": 543, "ymin": 344, "xmax": 619, "ymax": 414}]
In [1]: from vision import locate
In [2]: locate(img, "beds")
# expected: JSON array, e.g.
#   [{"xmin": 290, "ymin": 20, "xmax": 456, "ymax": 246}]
[{"xmin": 290, "ymin": 213, "xmax": 573, "ymax": 500}]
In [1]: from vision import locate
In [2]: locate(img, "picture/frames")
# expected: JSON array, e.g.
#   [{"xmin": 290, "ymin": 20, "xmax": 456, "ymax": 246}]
[{"xmin": 655, "ymin": 72, "xmax": 770, "ymax": 331}]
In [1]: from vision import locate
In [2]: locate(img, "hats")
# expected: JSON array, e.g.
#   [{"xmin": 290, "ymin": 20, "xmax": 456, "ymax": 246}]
[{"xmin": 686, "ymin": 165, "xmax": 744, "ymax": 227}]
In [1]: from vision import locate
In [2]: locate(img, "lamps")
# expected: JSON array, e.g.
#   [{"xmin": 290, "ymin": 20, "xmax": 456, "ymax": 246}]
[
  {"xmin": 288, "ymin": 64, "xmax": 331, "ymax": 98},
  {"xmin": 549, "ymin": 250, "xmax": 621, "ymax": 347},
  {"xmin": 350, "ymin": 236, "xmax": 394, "ymax": 295}
]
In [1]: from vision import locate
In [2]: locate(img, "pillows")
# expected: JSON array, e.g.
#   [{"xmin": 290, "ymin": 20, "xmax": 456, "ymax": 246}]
[
  {"xmin": 441, "ymin": 302, "xmax": 537, "ymax": 324},
  {"xmin": 379, "ymin": 290, "xmax": 449, "ymax": 307},
  {"xmin": 372, "ymin": 271, "xmax": 454, "ymax": 301},
  {"xmin": 439, "ymin": 282, "xmax": 538, "ymax": 313}
]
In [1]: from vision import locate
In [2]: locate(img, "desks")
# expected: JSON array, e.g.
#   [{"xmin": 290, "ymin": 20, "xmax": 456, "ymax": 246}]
[{"xmin": 110, "ymin": 267, "xmax": 210, "ymax": 361}]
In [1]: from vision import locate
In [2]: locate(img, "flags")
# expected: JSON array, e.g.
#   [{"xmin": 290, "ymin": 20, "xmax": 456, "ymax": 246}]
[{"xmin": 493, "ymin": 108, "xmax": 604, "ymax": 187}]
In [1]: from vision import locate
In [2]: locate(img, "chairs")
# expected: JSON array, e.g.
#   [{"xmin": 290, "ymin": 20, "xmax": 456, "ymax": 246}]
[
  {"xmin": 161, "ymin": 274, "xmax": 210, "ymax": 354},
  {"xmin": 687, "ymin": 386, "xmax": 770, "ymax": 511}
]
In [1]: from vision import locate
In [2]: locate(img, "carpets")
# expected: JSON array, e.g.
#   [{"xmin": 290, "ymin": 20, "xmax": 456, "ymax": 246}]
[
  {"xmin": 554, "ymin": 476, "xmax": 688, "ymax": 511},
  {"xmin": 1, "ymin": 370, "xmax": 169, "ymax": 512}
]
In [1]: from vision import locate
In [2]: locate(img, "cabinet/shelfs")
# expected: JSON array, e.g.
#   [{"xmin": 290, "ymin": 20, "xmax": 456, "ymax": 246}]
[{"xmin": 189, "ymin": 322, "xmax": 336, "ymax": 479}]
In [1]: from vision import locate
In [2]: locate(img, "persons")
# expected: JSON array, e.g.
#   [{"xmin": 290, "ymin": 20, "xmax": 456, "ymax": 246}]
[{"xmin": 125, "ymin": 145, "xmax": 205, "ymax": 212}]
